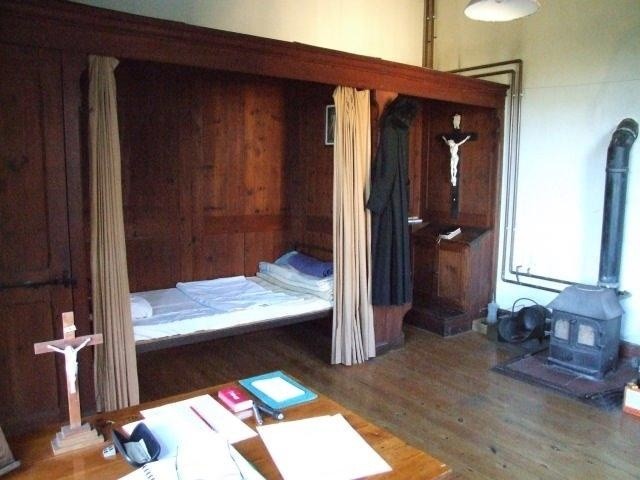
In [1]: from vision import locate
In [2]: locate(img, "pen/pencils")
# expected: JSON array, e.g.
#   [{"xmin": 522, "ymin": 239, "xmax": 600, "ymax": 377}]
[{"xmin": 190, "ymin": 406, "xmax": 216, "ymax": 432}]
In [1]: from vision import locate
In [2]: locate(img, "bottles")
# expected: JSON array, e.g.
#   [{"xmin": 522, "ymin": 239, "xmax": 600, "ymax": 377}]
[{"xmin": 487, "ymin": 300, "xmax": 500, "ymax": 323}]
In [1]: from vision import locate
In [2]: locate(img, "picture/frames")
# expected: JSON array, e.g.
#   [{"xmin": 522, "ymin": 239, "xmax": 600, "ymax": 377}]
[{"xmin": 325, "ymin": 104, "xmax": 337, "ymax": 146}]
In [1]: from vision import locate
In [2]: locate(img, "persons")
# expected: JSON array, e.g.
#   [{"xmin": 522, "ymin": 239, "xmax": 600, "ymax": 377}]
[
  {"xmin": 442, "ymin": 134, "xmax": 472, "ymax": 187},
  {"xmin": 46, "ymin": 337, "xmax": 92, "ymax": 395}
]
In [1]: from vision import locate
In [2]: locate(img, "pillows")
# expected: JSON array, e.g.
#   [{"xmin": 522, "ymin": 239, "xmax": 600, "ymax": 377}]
[{"xmin": 129, "ymin": 294, "xmax": 152, "ymax": 320}]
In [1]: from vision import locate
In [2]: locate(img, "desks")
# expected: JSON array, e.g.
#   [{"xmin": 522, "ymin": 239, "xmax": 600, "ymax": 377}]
[{"xmin": 0, "ymin": 371, "xmax": 452, "ymax": 480}]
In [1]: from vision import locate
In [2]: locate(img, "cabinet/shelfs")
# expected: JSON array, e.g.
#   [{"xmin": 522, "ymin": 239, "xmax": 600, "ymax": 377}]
[
  {"xmin": 409, "ymin": 234, "xmax": 493, "ymax": 312},
  {"xmin": 0, "ymin": 0, "xmax": 94, "ymax": 434}
]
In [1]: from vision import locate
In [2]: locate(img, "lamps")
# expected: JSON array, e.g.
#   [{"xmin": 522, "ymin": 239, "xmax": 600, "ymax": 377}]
[{"xmin": 463, "ymin": 0, "xmax": 540, "ymax": 22}]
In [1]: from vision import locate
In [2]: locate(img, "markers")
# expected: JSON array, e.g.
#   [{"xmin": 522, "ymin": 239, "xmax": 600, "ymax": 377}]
[
  {"xmin": 252, "ymin": 402, "xmax": 263, "ymax": 424},
  {"xmin": 257, "ymin": 404, "xmax": 284, "ymax": 420}
]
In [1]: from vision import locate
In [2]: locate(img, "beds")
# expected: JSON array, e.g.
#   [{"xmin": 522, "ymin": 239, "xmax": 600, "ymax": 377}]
[{"xmin": 131, "ymin": 253, "xmax": 332, "ymax": 355}]
